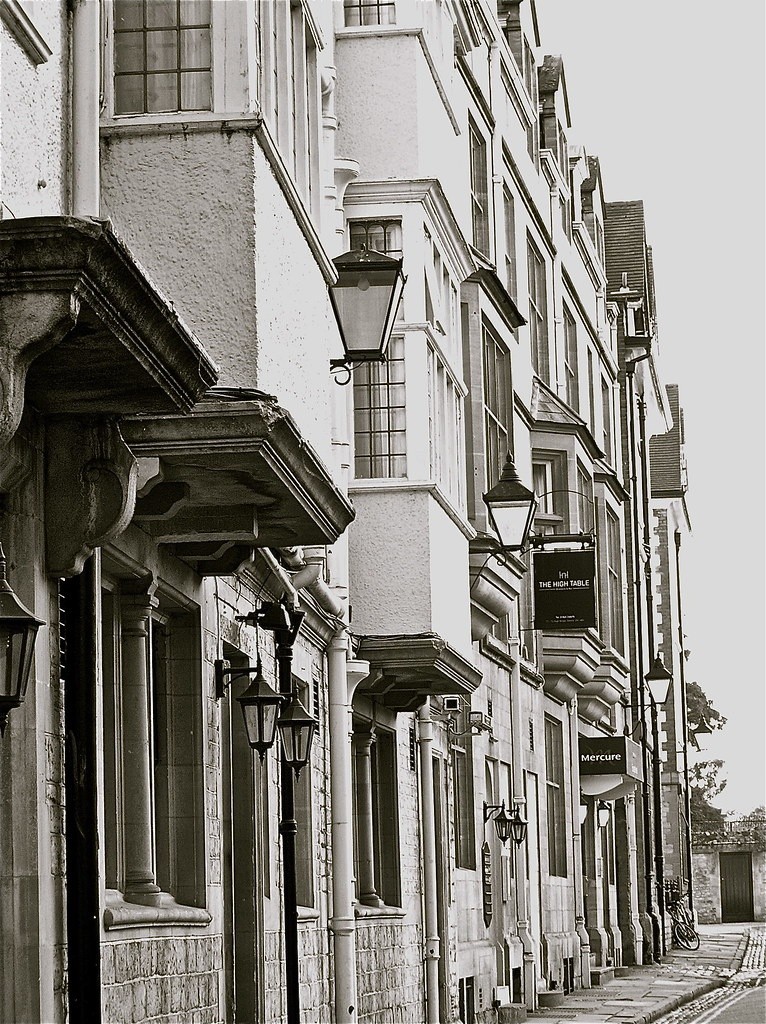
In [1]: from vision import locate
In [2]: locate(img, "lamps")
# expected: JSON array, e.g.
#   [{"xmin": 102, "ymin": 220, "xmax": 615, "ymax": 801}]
[
  {"xmin": 622, "ymin": 651, "xmax": 674, "ymax": 735},
  {"xmin": 597, "ymin": 799, "xmax": 611, "ymax": 827},
  {"xmin": 494, "ymin": 799, "xmax": 514, "ymax": 847},
  {"xmin": 236, "ymin": 653, "xmax": 286, "ymax": 767},
  {"xmin": 510, "ymin": 803, "xmax": 529, "ymax": 849},
  {"xmin": 469, "ymin": 450, "xmax": 540, "ymax": 589},
  {"xmin": 329, "ymin": 242, "xmax": 407, "ymax": 385},
  {"xmin": 275, "ymin": 680, "xmax": 317, "ymax": 782}
]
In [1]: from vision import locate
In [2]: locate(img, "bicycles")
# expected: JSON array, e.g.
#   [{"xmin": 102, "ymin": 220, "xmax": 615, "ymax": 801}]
[{"xmin": 669, "ymin": 898, "xmax": 705, "ymax": 950}]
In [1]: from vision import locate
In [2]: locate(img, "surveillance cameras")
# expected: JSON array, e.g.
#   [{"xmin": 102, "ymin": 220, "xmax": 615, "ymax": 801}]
[
  {"xmin": 443, "ymin": 697, "xmax": 463, "ymax": 713},
  {"xmin": 467, "ymin": 711, "xmax": 494, "ymax": 732}
]
